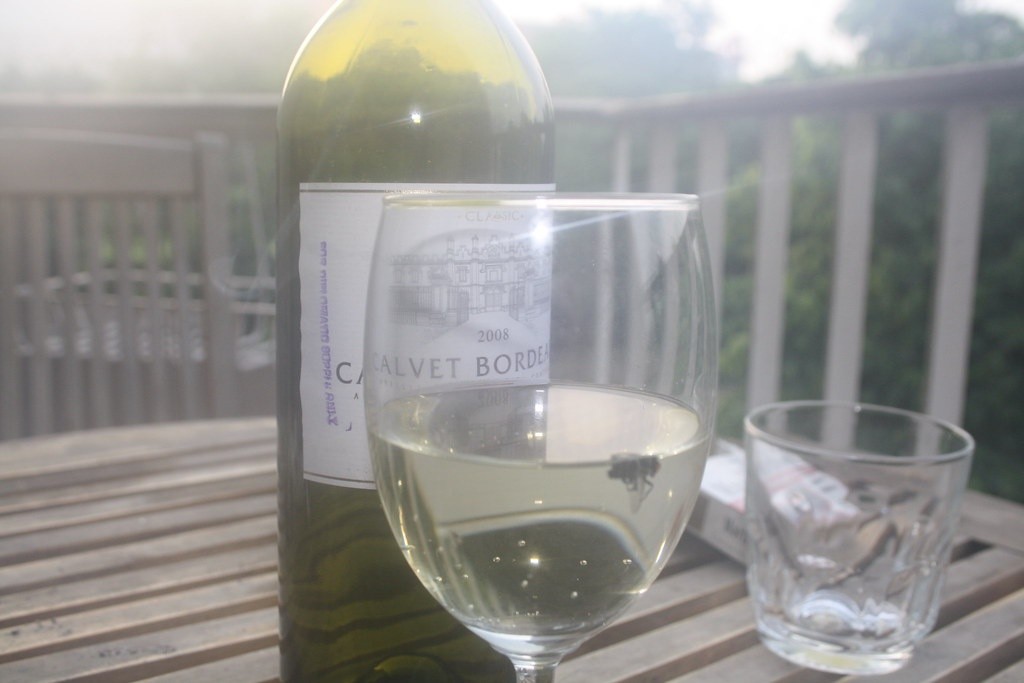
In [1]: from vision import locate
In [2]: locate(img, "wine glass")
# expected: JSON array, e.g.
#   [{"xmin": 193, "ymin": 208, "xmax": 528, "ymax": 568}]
[{"xmin": 364, "ymin": 192, "xmax": 719, "ymax": 683}]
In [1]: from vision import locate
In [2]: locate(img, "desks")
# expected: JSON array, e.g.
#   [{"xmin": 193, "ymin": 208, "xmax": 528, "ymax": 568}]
[{"xmin": 0, "ymin": 414, "xmax": 1024, "ymax": 683}]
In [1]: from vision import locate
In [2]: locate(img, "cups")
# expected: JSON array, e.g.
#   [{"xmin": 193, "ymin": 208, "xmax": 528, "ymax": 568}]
[{"xmin": 742, "ymin": 399, "xmax": 975, "ymax": 676}]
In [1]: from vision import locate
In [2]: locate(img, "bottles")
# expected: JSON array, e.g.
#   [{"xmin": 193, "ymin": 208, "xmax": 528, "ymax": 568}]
[{"xmin": 276, "ymin": 0, "xmax": 556, "ymax": 683}]
